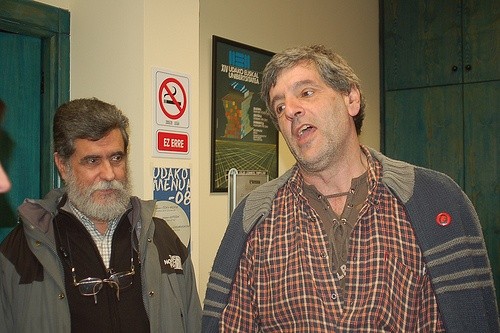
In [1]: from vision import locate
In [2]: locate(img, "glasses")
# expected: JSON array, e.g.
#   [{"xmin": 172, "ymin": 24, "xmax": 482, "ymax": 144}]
[{"xmin": 66, "ymin": 229, "xmax": 137, "ymax": 304}]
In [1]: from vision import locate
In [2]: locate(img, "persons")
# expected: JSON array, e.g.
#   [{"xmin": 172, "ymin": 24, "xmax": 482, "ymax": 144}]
[
  {"xmin": 201, "ymin": 44, "xmax": 500, "ymax": 333},
  {"xmin": 0, "ymin": 97, "xmax": 203, "ymax": 333}
]
